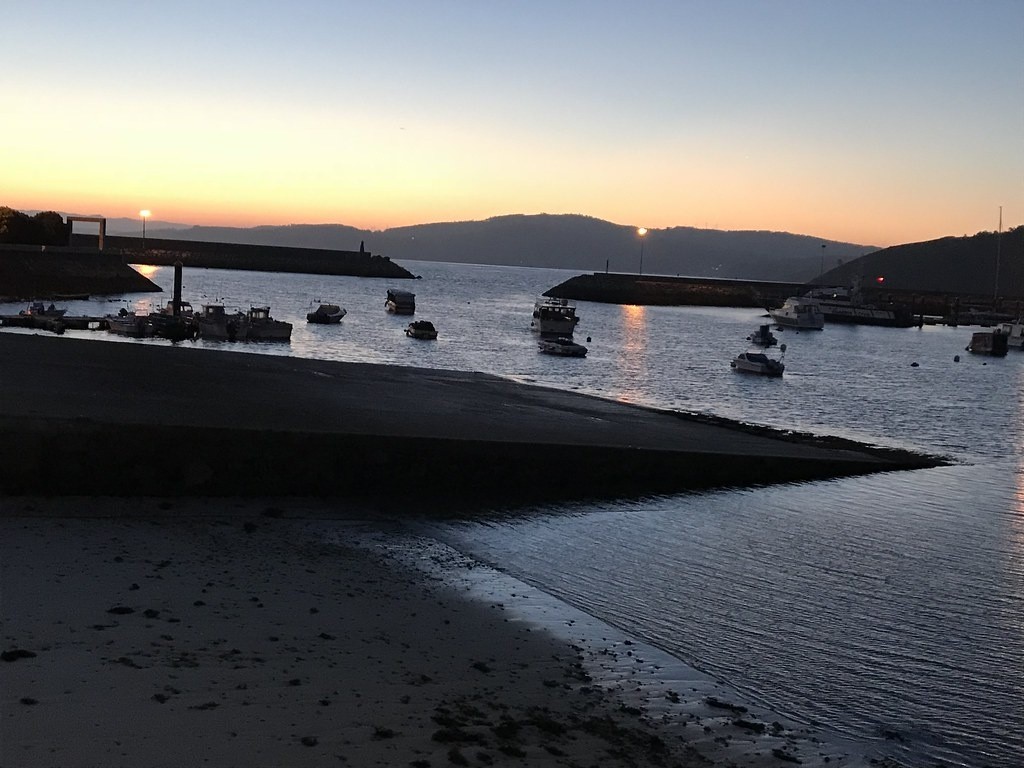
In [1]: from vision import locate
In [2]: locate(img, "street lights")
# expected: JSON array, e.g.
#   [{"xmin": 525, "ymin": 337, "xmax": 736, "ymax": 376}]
[{"xmin": 820, "ymin": 244, "xmax": 826, "ymax": 276}]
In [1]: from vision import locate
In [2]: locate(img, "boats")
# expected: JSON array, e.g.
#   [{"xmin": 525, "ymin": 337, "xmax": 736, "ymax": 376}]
[
  {"xmin": 749, "ymin": 325, "xmax": 777, "ymax": 346},
  {"xmin": 0, "ymin": 258, "xmax": 293, "ymax": 342},
  {"xmin": 530, "ymin": 292, "xmax": 580, "ymax": 334},
  {"xmin": 963, "ymin": 317, "xmax": 1024, "ymax": 358},
  {"xmin": 769, "ymin": 290, "xmax": 825, "ymax": 329},
  {"xmin": 802, "ymin": 286, "xmax": 897, "ymax": 324},
  {"xmin": 306, "ymin": 304, "xmax": 348, "ymax": 325},
  {"xmin": 404, "ymin": 320, "xmax": 439, "ymax": 340},
  {"xmin": 536, "ymin": 338, "xmax": 589, "ymax": 358},
  {"xmin": 384, "ymin": 288, "xmax": 416, "ymax": 315},
  {"xmin": 732, "ymin": 341, "xmax": 787, "ymax": 376}
]
